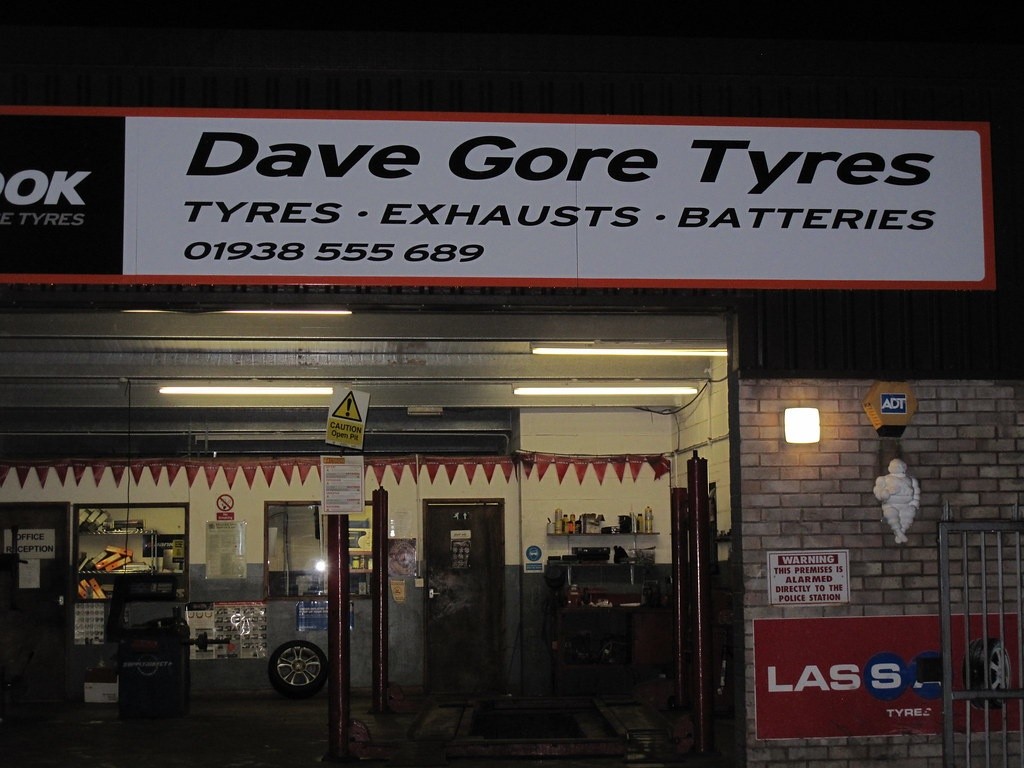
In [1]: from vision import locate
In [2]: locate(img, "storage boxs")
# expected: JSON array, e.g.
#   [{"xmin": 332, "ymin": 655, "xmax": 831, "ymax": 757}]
[{"xmin": 73, "ymin": 508, "xmax": 144, "ymax": 704}]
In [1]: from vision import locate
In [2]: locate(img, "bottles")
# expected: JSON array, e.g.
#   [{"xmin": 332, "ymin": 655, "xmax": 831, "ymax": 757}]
[
  {"xmin": 547, "ymin": 508, "xmax": 582, "ymax": 535},
  {"xmin": 630, "ymin": 506, "xmax": 652, "ymax": 533},
  {"xmin": 353, "ymin": 555, "xmax": 373, "ymax": 570}
]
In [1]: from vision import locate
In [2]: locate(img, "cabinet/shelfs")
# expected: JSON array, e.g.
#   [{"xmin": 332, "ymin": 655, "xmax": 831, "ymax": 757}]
[
  {"xmin": 547, "ymin": 533, "xmax": 660, "ymax": 585},
  {"xmin": 72, "ymin": 502, "xmax": 189, "ymax": 710},
  {"xmin": 550, "ymin": 606, "xmax": 675, "ymax": 697}
]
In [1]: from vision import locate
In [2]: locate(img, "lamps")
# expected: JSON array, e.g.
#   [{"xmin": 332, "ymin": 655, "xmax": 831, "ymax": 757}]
[
  {"xmin": 512, "ymin": 382, "xmax": 699, "ymax": 396},
  {"xmin": 529, "ymin": 342, "xmax": 728, "ymax": 357}
]
